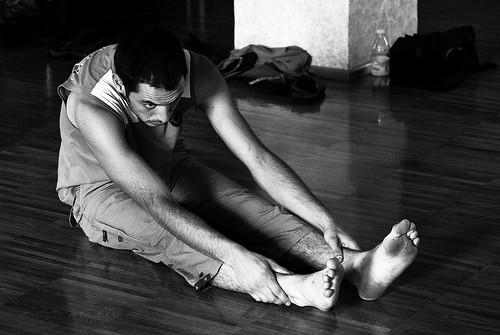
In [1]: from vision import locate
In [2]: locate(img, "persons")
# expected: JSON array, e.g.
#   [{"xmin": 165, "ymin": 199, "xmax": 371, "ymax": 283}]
[{"xmin": 56, "ymin": 29, "xmax": 420, "ymax": 312}]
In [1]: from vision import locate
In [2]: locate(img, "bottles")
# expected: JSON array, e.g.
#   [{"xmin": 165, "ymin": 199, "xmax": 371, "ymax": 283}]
[{"xmin": 371, "ymin": 29, "xmax": 390, "ymax": 77}]
